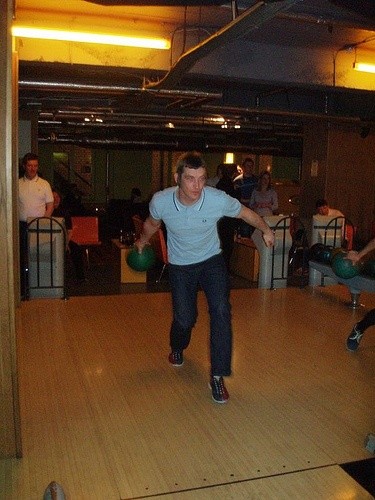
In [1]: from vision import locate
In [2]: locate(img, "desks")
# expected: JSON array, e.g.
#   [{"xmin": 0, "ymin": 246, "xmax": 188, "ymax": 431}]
[
  {"xmin": 111, "ymin": 238, "xmax": 147, "ymax": 283},
  {"xmin": 230, "ymin": 237, "xmax": 259, "ymax": 283}
]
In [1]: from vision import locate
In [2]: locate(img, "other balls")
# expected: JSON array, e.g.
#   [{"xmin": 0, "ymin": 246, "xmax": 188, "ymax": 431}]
[
  {"xmin": 333, "ymin": 253, "xmax": 362, "ymax": 278},
  {"xmin": 126, "ymin": 246, "xmax": 157, "ymax": 271},
  {"xmin": 309, "ymin": 242, "xmax": 358, "ymax": 267}
]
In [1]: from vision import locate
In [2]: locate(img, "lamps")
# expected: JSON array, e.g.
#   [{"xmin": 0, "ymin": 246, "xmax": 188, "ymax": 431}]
[
  {"xmin": 353, "ymin": 63, "xmax": 375, "ymax": 74},
  {"xmin": 12, "ymin": 25, "xmax": 171, "ymax": 50}
]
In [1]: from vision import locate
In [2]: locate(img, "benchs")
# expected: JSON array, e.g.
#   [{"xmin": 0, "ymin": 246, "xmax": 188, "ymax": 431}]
[
  {"xmin": 68, "ymin": 215, "xmax": 101, "ymax": 274},
  {"xmin": 133, "ymin": 215, "xmax": 168, "ymax": 284}
]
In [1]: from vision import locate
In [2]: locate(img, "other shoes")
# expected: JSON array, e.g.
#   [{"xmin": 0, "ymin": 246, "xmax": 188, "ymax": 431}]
[
  {"xmin": 207, "ymin": 376, "xmax": 230, "ymax": 403},
  {"xmin": 168, "ymin": 348, "xmax": 185, "ymax": 367},
  {"xmin": 344, "ymin": 321, "xmax": 364, "ymax": 350}
]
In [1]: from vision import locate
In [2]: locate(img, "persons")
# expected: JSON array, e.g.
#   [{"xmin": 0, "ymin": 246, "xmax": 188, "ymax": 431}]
[
  {"xmin": 18, "ymin": 153, "xmax": 72, "ymax": 296},
  {"xmin": 63, "ymin": 184, "xmax": 82, "ymax": 206},
  {"xmin": 130, "ymin": 188, "xmax": 143, "ymax": 203},
  {"xmin": 343, "ymin": 239, "xmax": 375, "ymax": 353},
  {"xmin": 133, "ymin": 151, "xmax": 274, "ymax": 404},
  {"xmin": 208, "ymin": 158, "xmax": 344, "ymax": 281}
]
